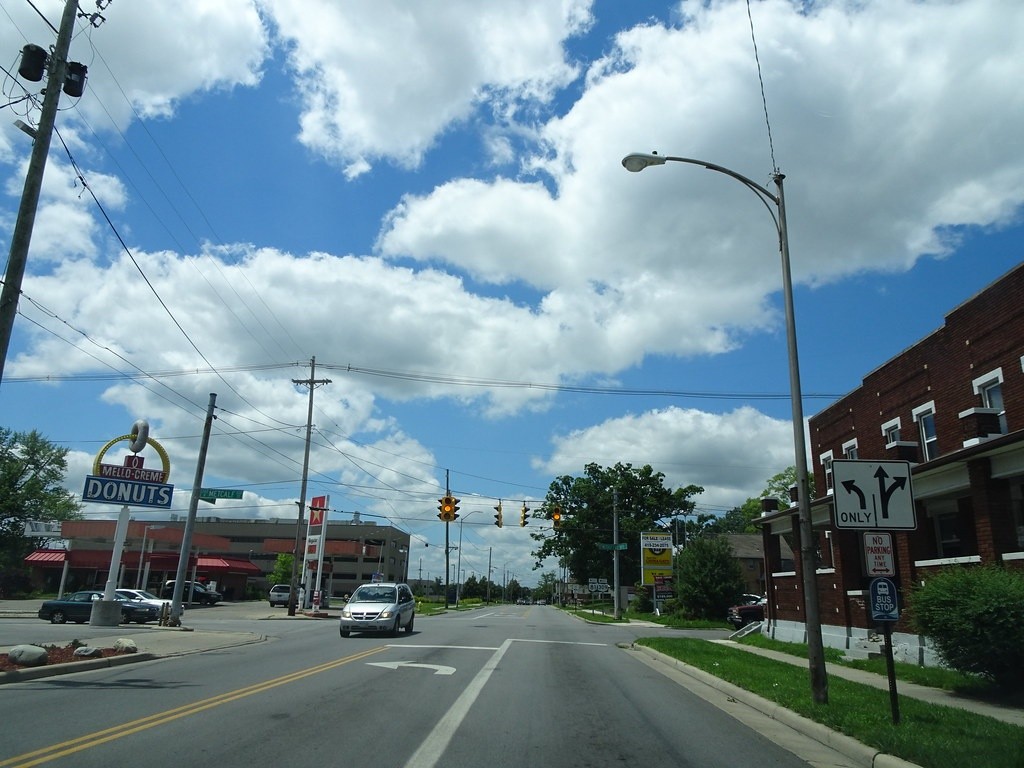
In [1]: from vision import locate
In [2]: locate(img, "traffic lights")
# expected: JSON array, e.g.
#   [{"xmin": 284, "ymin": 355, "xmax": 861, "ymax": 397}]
[
  {"xmin": 554, "ymin": 507, "xmax": 561, "ymax": 528},
  {"xmin": 493, "ymin": 506, "xmax": 502, "ymax": 529},
  {"xmin": 437, "ymin": 497, "xmax": 460, "ymax": 522},
  {"xmin": 520, "ymin": 506, "xmax": 530, "ymax": 528}
]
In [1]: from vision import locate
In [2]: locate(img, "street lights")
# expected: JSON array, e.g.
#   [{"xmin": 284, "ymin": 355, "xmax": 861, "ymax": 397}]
[
  {"xmin": 622, "ymin": 150, "xmax": 830, "ymax": 703},
  {"xmin": 452, "ymin": 564, "xmax": 481, "ymax": 583},
  {"xmin": 503, "ymin": 560, "xmax": 512, "ymax": 599},
  {"xmin": 456, "ymin": 511, "xmax": 484, "ymax": 608}
]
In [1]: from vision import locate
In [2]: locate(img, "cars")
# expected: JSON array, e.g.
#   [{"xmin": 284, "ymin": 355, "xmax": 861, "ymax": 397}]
[
  {"xmin": 268, "ymin": 584, "xmax": 305, "ymax": 608},
  {"xmin": 38, "ymin": 591, "xmax": 160, "ymax": 624},
  {"xmin": 516, "ymin": 597, "xmax": 547, "ymax": 606},
  {"xmin": 727, "ymin": 593, "xmax": 767, "ymax": 629},
  {"xmin": 119, "ymin": 590, "xmax": 185, "ymax": 619},
  {"xmin": 162, "ymin": 581, "xmax": 222, "ymax": 607}
]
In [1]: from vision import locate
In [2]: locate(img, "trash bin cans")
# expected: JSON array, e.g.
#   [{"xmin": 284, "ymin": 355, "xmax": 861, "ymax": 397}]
[{"xmin": 562, "ymin": 600, "xmax": 567, "ymax": 607}]
